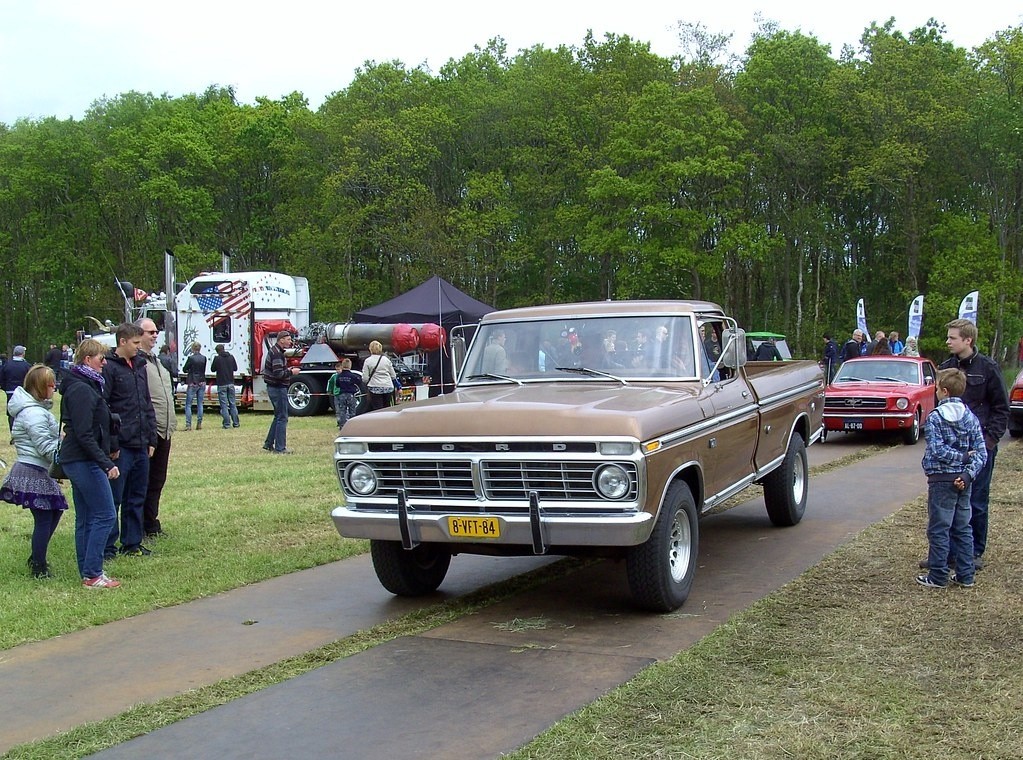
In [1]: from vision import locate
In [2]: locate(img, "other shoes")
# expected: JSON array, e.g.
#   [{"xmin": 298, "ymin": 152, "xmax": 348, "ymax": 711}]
[
  {"xmin": 181, "ymin": 424, "xmax": 202, "ymax": 431},
  {"xmin": 262, "ymin": 446, "xmax": 293, "ymax": 455},
  {"xmin": 146, "ymin": 531, "xmax": 168, "ymax": 540},
  {"xmin": 920, "ymin": 560, "xmax": 955, "ymax": 569},
  {"xmin": 973, "ymin": 553, "xmax": 984, "ymax": 569},
  {"xmin": 105, "ymin": 547, "xmax": 118, "ymax": 563}
]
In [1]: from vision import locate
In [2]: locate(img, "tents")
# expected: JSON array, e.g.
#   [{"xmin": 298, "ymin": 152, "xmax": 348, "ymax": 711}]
[{"xmin": 352, "ymin": 275, "xmax": 500, "ymax": 398}]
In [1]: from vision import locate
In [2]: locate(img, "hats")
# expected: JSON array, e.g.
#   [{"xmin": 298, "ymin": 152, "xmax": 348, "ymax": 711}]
[{"xmin": 13, "ymin": 345, "xmax": 26, "ymax": 356}]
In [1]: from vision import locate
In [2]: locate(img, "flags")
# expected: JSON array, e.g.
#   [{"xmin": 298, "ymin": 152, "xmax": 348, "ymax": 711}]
[{"xmin": 134, "ymin": 288, "xmax": 148, "ymax": 301}]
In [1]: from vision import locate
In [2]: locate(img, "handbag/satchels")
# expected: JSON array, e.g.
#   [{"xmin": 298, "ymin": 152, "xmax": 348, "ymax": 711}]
[{"xmin": 48, "ymin": 451, "xmax": 71, "ymax": 479}]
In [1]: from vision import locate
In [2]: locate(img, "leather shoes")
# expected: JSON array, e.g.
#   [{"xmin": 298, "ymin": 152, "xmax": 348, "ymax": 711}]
[{"xmin": 120, "ymin": 544, "xmax": 151, "ymax": 557}]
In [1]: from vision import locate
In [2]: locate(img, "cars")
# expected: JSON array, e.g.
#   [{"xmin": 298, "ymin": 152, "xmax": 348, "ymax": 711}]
[{"xmin": 828, "ymin": 355, "xmax": 940, "ymax": 443}]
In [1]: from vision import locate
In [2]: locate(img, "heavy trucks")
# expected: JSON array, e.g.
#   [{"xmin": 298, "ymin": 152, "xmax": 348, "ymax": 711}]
[{"xmin": 75, "ymin": 271, "xmax": 447, "ymax": 416}]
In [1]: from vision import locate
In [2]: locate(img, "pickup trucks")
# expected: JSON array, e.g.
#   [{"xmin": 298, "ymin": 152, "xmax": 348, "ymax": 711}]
[{"xmin": 327, "ymin": 296, "xmax": 826, "ymax": 614}]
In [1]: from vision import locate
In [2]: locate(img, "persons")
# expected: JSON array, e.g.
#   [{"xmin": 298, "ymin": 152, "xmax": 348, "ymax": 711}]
[
  {"xmin": 58, "ymin": 339, "xmax": 122, "ymax": 590},
  {"xmin": 480, "ymin": 330, "xmax": 507, "ymax": 374},
  {"xmin": 100, "ymin": 324, "xmax": 157, "ymax": 559},
  {"xmin": 0, "ymin": 343, "xmax": 34, "ymax": 447},
  {"xmin": 751, "ymin": 337, "xmax": 783, "ymax": 361},
  {"xmin": 133, "ymin": 318, "xmax": 178, "ymax": 539},
  {"xmin": 543, "ymin": 319, "xmax": 720, "ymax": 382},
  {"xmin": 822, "ymin": 333, "xmax": 838, "ymax": 386},
  {"xmin": 157, "ymin": 341, "xmax": 177, "ymax": 406},
  {"xmin": 325, "ymin": 359, "xmax": 362, "ymax": 431},
  {"xmin": 42, "ymin": 342, "xmax": 77, "ymax": 392},
  {"xmin": 912, "ymin": 368, "xmax": 988, "ymax": 589},
  {"xmin": 705, "ymin": 324, "xmax": 724, "ymax": 363},
  {"xmin": 153, "ymin": 312, "xmax": 164, "ymax": 330},
  {"xmin": 211, "ymin": 345, "xmax": 241, "ymax": 429},
  {"xmin": 918, "ymin": 319, "xmax": 1010, "ymax": 570},
  {"xmin": 818, "ymin": 361, "xmax": 824, "ymax": 372},
  {"xmin": 362, "ymin": 339, "xmax": 402, "ymax": 410},
  {"xmin": 0, "ymin": 364, "xmax": 68, "ymax": 579},
  {"xmin": 843, "ymin": 330, "xmax": 919, "ymax": 363},
  {"xmin": 263, "ymin": 331, "xmax": 301, "ymax": 453}
]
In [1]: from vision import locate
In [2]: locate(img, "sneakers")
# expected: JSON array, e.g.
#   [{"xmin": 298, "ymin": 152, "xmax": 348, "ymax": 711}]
[
  {"xmin": 915, "ymin": 575, "xmax": 947, "ymax": 589},
  {"xmin": 949, "ymin": 574, "xmax": 975, "ymax": 587},
  {"xmin": 28, "ymin": 560, "xmax": 56, "ymax": 582},
  {"xmin": 81, "ymin": 576, "xmax": 121, "ymax": 589}
]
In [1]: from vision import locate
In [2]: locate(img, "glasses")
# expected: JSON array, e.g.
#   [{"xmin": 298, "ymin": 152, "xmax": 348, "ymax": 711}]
[
  {"xmin": 99, "ymin": 355, "xmax": 105, "ymax": 363},
  {"xmin": 662, "ymin": 332, "xmax": 668, "ymax": 335},
  {"xmin": 48, "ymin": 383, "xmax": 55, "ymax": 388},
  {"xmin": 144, "ymin": 330, "xmax": 160, "ymax": 335}
]
